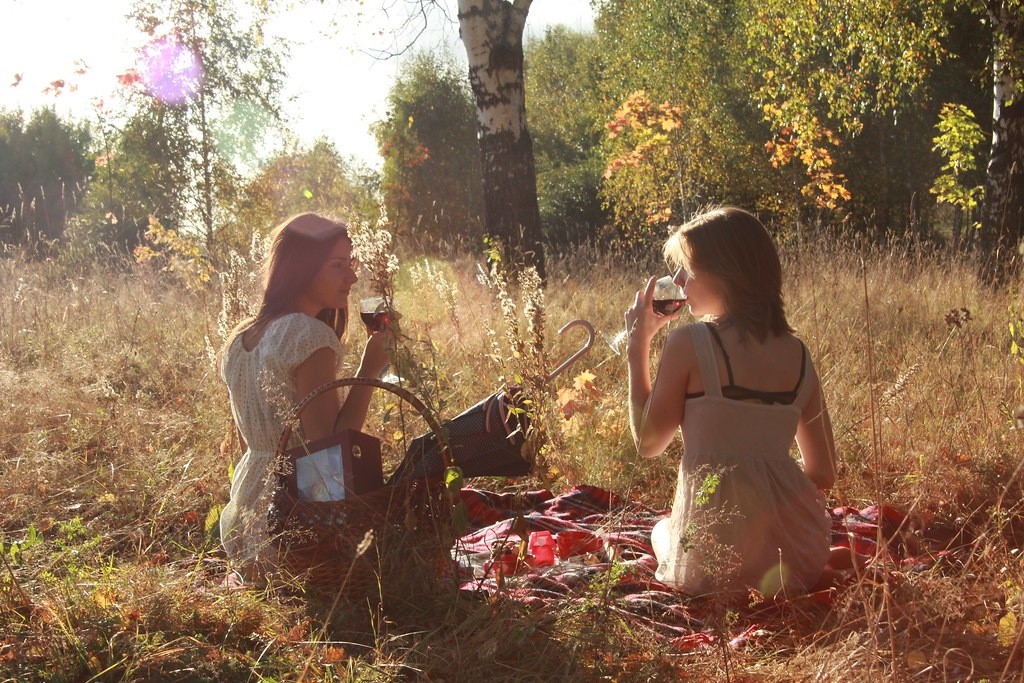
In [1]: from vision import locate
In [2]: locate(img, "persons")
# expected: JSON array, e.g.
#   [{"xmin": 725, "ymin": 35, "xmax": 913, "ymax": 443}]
[
  {"xmin": 624, "ymin": 208, "xmax": 838, "ymax": 599},
  {"xmin": 219, "ymin": 213, "xmax": 395, "ymax": 582}
]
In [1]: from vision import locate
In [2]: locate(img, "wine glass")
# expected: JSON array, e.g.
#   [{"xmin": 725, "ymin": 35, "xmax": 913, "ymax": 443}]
[
  {"xmin": 599, "ymin": 274, "xmax": 688, "ymax": 357},
  {"xmin": 360, "ymin": 297, "xmax": 406, "ymax": 385}
]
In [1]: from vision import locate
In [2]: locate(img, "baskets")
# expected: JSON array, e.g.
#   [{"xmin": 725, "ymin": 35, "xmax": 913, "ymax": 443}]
[{"xmin": 264, "ymin": 376, "xmax": 456, "ymax": 607}]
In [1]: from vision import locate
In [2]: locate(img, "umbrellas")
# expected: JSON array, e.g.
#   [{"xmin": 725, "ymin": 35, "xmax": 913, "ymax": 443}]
[{"xmin": 384, "ymin": 319, "xmax": 595, "ymax": 487}]
